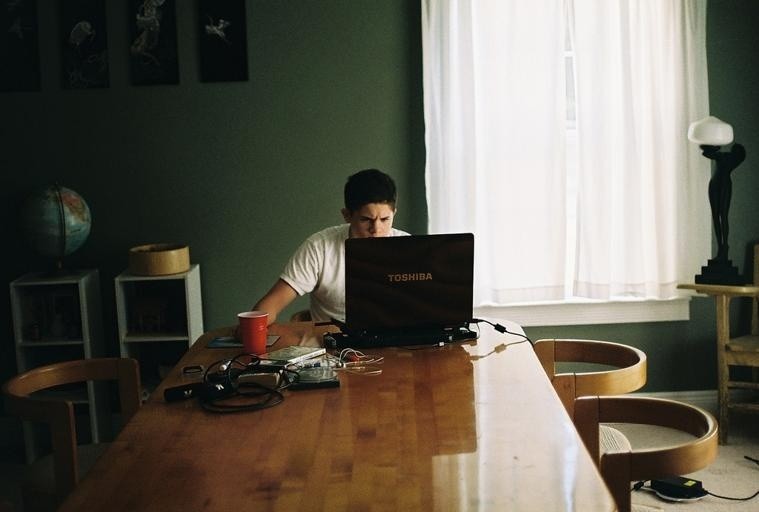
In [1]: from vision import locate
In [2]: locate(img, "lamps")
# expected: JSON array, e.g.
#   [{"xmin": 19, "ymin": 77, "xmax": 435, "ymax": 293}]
[{"xmin": 688, "ymin": 116, "xmax": 746, "ymax": 286}]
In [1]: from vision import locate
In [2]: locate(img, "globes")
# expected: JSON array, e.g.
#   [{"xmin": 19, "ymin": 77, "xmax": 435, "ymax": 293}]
[{"xmin": 17, "ymin": 180, "xmax": 95, "ymax": 279}]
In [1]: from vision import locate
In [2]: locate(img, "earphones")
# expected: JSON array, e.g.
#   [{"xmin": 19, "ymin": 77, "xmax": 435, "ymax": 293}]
[{"xmin": 336, "ymin": 360, "xmax": 342, "ymax": 367}]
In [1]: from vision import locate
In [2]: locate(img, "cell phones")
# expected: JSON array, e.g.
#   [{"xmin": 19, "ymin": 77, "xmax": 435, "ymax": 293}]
[{"xmin": 247, "ymin": 360, "xmax": 289, "ymax": 368}]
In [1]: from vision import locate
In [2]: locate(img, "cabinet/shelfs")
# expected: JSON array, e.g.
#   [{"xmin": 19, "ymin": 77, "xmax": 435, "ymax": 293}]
[
  {"xmin": 10, "ymin": 269, "xmax": 113, "ymax": 464},
  {"xmin": 114, "ymin": 263, "xmax": 204, "ymax": 402}
]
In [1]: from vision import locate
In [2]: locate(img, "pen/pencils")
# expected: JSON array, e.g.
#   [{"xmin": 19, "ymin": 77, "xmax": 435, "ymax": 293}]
[{"xmin": 333, "ymin": 350, "xmax": 360, "ymax": 362}]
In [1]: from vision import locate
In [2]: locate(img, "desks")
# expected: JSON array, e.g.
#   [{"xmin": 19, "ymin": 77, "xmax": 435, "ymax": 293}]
[
  {"xmin": 56, "ymin": 321, "xmax": 617, "ymax": 512},
  {"xmin": 676, "ymin": 284, "xmax": 759, "ymax": 446}
]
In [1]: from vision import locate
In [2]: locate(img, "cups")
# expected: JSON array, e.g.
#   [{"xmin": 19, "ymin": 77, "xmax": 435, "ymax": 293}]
[{"xmin": 237, "ymin": 311, "xmax": 270, "ymax": 355}]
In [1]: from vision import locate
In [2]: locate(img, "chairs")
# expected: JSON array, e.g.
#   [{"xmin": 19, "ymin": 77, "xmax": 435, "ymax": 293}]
[
  {"xmin": 533, "ymin": 339, "xmax": 648, "ymax": 454},
  {"xmin": 2, "ymin": 359, "xmax": 143, "ymax": 512},
  {"xmin": 575, "ymin": 394, "xmax": 717, "ymax": 512},
  {"xmin": 290, "ymin": 310, "xmax": 312, "ymax": 321}
]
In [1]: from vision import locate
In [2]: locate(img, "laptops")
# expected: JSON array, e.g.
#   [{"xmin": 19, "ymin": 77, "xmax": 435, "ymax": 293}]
[{"xmin": 330, "ymin": 232, "xmax": 474, "ymax": 348}]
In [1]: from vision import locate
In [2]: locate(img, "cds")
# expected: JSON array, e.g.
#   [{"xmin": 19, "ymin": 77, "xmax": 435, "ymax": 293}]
[{"xmin": 293, "ymin": 368, "xmax": 338, "ymax": 381}]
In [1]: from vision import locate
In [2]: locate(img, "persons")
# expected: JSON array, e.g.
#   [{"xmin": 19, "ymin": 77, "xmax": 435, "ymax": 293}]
[{"xmin": 232, "ymin": 168, "xmax": 413, "ymax": 343}]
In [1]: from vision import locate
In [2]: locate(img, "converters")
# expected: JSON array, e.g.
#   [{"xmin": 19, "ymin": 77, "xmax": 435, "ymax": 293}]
[{"xmin": 649, "ymin": 476, "xmax": 703, "ymax": 498}]
